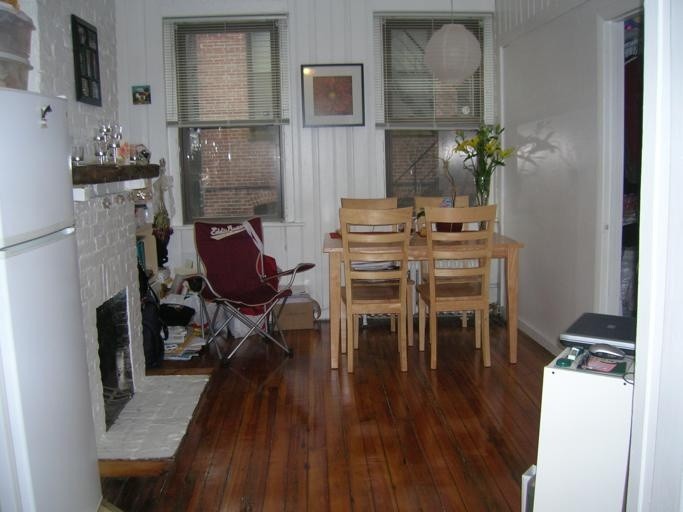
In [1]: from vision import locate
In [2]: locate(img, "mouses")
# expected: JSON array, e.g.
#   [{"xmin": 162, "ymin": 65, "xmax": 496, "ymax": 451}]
[{"xmin": 589, "ymin": 343, "xmax": 626, "ymax": 359}]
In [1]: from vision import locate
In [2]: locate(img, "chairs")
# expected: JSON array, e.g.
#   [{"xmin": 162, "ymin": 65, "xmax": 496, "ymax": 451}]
[
  {"xmin": 340, "ymin": 206, "xmax": 413, "ymax": 374},
  {"xmin": 414, "ymin": 195, "xmax": 481, "ymax": 327},
  {"xmin": 340, "ymin": 197, "xmax": 415, "ymax": 345},
  {"xmin": 183, "ymin": 217, "xmax": 316, "ymax": 368},
  {"xmin": 416, "ymin": 203, "xmax": 498, "ymax": 370}
]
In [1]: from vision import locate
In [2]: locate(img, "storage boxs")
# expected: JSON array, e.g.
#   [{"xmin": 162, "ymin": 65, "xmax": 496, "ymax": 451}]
[
  {"xmin": 0, "ymin": 1, "xmax": 36, "ymax": 59},
  {"xmin": 136, "ymin": 223, "xmax": 158, "ymax": 272},
  {"xmin": 273, "ymin": 302, "xmax": 314, "ymax": 330},
  {"xmin": 0, "ymin": 52, "xmax": 33, "ymax": 90}
]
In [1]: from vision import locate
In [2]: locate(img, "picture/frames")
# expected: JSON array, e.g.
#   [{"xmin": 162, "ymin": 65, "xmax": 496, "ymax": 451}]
[{"xmin": 301, "ymin": 63, "xmax": 365, "ymax": 128}]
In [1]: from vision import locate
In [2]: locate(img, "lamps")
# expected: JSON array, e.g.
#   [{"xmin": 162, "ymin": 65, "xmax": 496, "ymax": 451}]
[{"xmin": 423, "ymin": 0, "xmax": 480, "ymax": 85}]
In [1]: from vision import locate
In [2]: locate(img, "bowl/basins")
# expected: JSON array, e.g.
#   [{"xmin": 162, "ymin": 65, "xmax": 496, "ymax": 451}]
[{"xmin": 159, "ymin": 303, "xmax": 195, "ymax": 327}]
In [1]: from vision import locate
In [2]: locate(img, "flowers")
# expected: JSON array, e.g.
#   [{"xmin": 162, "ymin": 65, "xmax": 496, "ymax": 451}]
[{"xmin": 455, "ymin": 124, "xmax": 515, "ymax": 230}]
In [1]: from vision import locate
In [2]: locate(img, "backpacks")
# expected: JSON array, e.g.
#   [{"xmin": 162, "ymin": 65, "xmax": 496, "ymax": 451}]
[{"xmin": 139, "ymin": 259, "xmax": 168, "ymax": 369}]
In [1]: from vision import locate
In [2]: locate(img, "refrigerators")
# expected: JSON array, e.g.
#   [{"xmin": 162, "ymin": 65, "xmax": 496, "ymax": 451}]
[{"xmin": 0, "ymin": 86, "xmax": 106, "ymax": 512}]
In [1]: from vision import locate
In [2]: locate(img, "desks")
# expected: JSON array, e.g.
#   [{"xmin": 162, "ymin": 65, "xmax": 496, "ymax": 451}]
[
  {"xmin": 521, "ymin": 348, "xmax": 634, "ymax": 512},
  {"xmin": 324, "ymin": 231, "xmax": 524, "ymax": 369}
]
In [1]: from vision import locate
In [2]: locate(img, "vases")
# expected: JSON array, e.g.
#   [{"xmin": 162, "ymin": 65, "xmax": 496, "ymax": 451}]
[
  {"xmin": 436, "ymin": 223, "xmax": 462, "ymax": 232},
  {"xmin": 474, "ymin": 177, "xmax": 490, "ymax": 244}
]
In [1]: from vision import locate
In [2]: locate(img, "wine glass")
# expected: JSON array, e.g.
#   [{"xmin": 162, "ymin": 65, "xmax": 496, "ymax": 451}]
[{"xmin": 70, "ymin": 120, "xmax": 122, "ymax": 168}]
